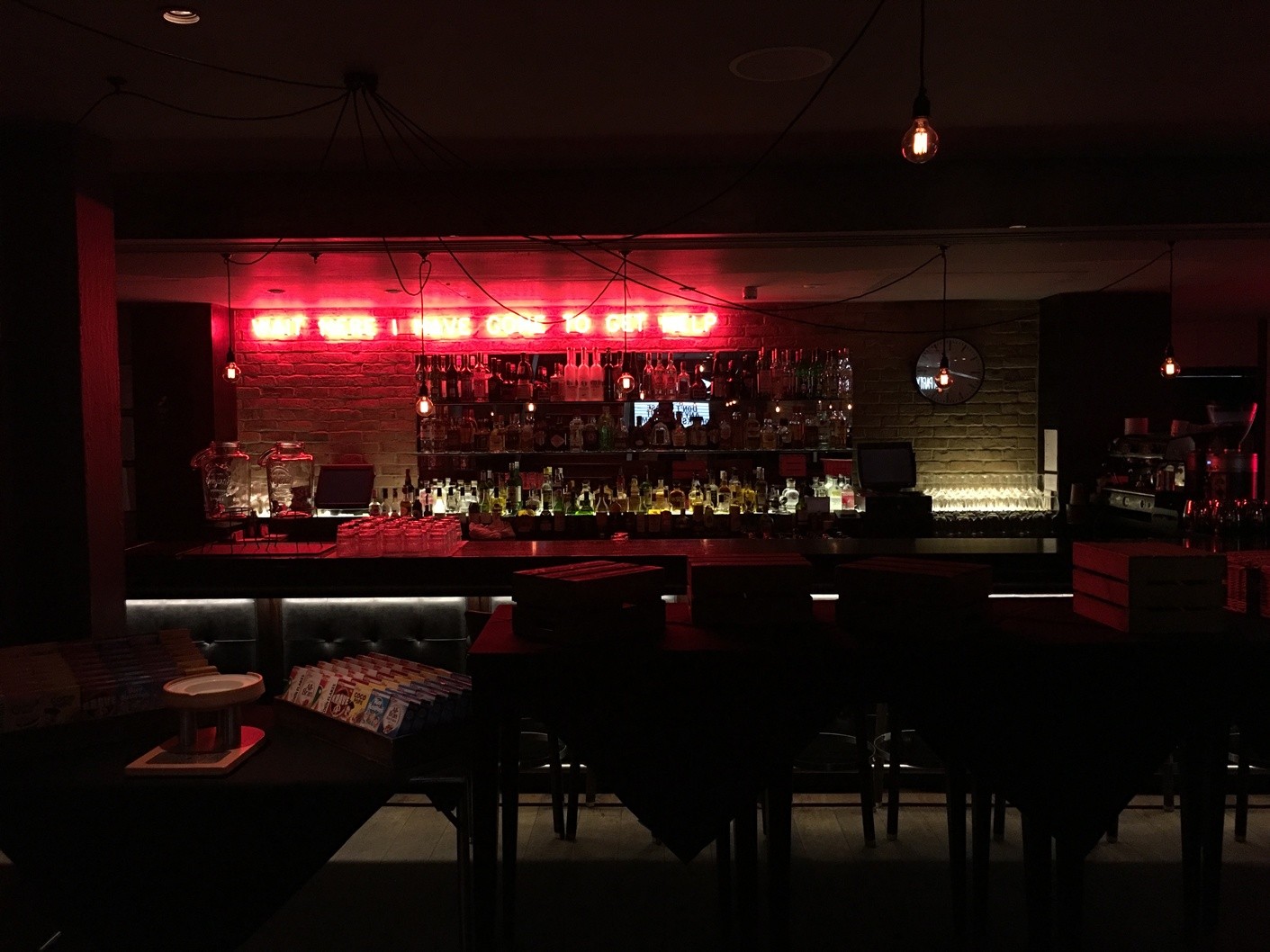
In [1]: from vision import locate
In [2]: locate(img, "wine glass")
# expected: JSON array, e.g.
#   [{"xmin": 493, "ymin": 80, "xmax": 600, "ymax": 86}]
[{"xmin": 920, "ymin": 475, "xmax": 1058, "ymax": 539}]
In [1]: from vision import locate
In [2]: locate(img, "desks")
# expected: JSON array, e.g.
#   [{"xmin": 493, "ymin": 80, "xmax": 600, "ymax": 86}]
[{"xmin": 0, "ymin": 596, "xmax": 1270, "ymax": 952}]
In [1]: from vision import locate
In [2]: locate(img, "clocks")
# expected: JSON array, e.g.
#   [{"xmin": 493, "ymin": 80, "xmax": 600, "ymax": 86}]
[{"xmin": 915, "ymin": 338, "xmax": 984, "ymax": 405}]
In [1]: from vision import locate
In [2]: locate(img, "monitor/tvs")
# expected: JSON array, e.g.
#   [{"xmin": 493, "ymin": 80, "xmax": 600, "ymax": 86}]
[
  {"xmin": 855, "ymin": 441, "xmax": 916, "ymax": 492},
  {"xmin": 315, "ymin": 465, "xmax": 375, "ymax": 509}
]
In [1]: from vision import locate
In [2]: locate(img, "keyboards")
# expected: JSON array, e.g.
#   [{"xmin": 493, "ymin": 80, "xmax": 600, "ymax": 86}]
[{"xmin": 873, "ymin": 491, "xmax": 920, "ymax": 497}]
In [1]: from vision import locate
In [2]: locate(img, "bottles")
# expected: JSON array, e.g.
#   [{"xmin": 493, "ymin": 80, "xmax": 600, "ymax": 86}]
[
  {"xmin": 368, "ymin": 462, "xmax": 865, "ymax": 543},
  {"xmin": 191, "ymin": 441, "xmax": 250, "ymax": 519},
  {"xmin": 417, "ymin": 345, "xmax": 853, "ymax": 450},
  {"xmin": 1183, "ymin": 498, "xmax": 1269, "ymax": 553},
  {"xmin": 258, "ymin": 441, "xmax": 315, "ymax": 519}
]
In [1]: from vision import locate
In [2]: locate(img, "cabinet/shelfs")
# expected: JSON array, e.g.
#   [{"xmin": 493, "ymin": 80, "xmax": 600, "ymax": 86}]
[{"xmin": 414, "ymin": 353, "xmax": 854, "ymax": 488}]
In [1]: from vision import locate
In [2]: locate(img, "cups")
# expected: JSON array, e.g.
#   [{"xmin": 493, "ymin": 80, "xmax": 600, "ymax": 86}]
[
  {"xmin": 217, "ymin": 703, "xmax": 240, "ymax": 746},
  {"xmin": 336, "ymin": 514, "xmax": 462, "ymax": 557},
  {"xmin": 1125, "ymin": 417, "xmax": 1148, "ymax": 434},
  {"xmin": 179, "ymin": 711, "xmax": 196, "ymax": 751}
]
